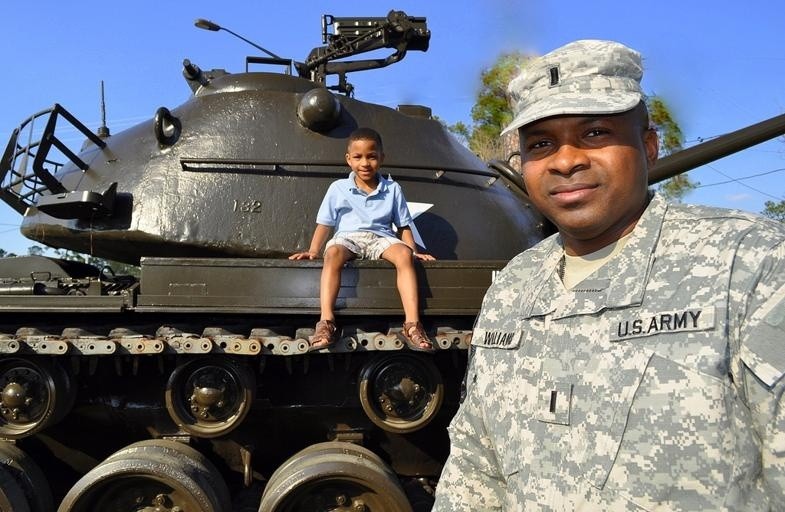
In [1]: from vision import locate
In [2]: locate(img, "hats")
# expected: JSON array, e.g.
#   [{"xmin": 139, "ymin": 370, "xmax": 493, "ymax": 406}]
[{"xmin": 499, "ymin": 40, "xmax": 643, "ymax": 134}]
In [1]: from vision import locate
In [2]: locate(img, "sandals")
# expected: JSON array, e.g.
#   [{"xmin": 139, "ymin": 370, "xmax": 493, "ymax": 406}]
[
  {"xmin": 308, "ymin": 319, "xmax": 340, "ymax": 353},
  {"xmin": 397, "ymin": 322, "xmax": 436, "ymax": 353}
]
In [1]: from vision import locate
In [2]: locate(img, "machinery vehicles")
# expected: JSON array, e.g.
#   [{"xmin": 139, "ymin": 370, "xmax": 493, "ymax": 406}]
[{"xmin": 0, "ymin": 12, "xmax": 784, "ymax": 511}]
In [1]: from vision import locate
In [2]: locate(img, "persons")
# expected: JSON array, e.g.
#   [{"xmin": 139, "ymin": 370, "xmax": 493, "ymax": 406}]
[
  {"xmin": 288, "ymin": 127, "xmax": 436, "ymax": 351},
  {"xmin": 432, "ymin": 39, "xmax": 785, "ymax": 512}
]
